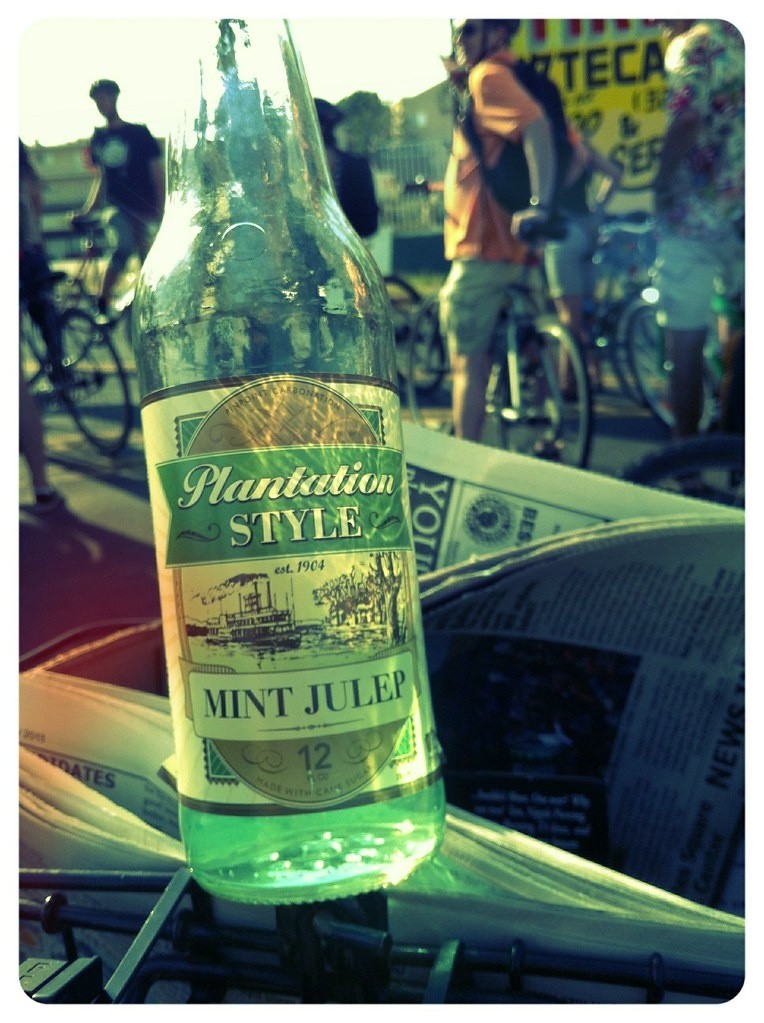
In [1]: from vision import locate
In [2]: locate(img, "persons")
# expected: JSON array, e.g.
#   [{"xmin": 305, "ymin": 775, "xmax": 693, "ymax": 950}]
[
  {"xmin": 62, "ymin": 79, "xmax": 167, "ymax": 327},
  {"xmin": 436, "ymin": 20, "xmax": 624, "ymax": 445},
  {"xmin": 19, "ymin": 133, "xmax": 75, "ymax": 520},
  {"xmin": 654, "ymin": 20, "xmax": 745, "ymax": 505},
  {"xmin": 312, "ymin": 95, "xmax": 382, "ymax": 238}
]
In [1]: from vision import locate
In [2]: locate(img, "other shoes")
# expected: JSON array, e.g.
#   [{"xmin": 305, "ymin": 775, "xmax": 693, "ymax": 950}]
[{"xmin": 34, "ymin": 489, "xmax": 58, "ymax": 513}]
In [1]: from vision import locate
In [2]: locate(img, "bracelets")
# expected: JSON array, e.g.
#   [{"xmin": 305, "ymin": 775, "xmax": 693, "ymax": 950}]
[{"xmin": 529, "ymin": 200, "xmax": 550, "ymax": 212}]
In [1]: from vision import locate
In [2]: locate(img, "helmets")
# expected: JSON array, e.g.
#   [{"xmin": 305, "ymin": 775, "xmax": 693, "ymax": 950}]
[
  {"xmin": 90, "ymin": 79, "xmax": 120, "ymax": 97},
  {"xmin": 313, "ymin": 97, "xmax": 342, "ymax": 127},
  {"xmin": 500, "ymin": 19, "xmax": 521, "ymax": 33}
]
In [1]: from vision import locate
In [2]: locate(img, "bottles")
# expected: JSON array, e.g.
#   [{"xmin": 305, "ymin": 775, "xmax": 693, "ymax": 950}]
[{"xmin": 130, "ymin": 18, "xmax": 448, "ymax": 908}]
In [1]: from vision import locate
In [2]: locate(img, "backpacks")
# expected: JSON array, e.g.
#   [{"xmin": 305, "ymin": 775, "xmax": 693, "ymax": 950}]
[
  {"xmin": 462, "ymin": 59, "xmax": 574, "ymax": 214},
  {"xmin": 327, "ymin": 148, "xmax": 377, "ymax": 236}
]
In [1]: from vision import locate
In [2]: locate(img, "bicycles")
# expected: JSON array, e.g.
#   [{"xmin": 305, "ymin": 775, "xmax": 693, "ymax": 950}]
[
  {"xmin": 28, "ymin": 218, "xmax": 139, "ymax": 374},
  {"xmin": 19, "ymin": 270, "xmax": 135, "ymax": 449},
  {"xmin": 581, "ymin": 192, "xmax": 742, "ymax": 441},
  {"xmin": 403, "ymin": 206, "xmax": 594, "ymax": 473},
  {"xmin": 386, "ymin": 276, "xmax": 447, "ymax": 396}
]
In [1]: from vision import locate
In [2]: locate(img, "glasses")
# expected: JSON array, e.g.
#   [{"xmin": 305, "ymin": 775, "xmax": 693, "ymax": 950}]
[{"xmin": 455, "ymin": 23, "xmax": 480, "ymax": 40}]
